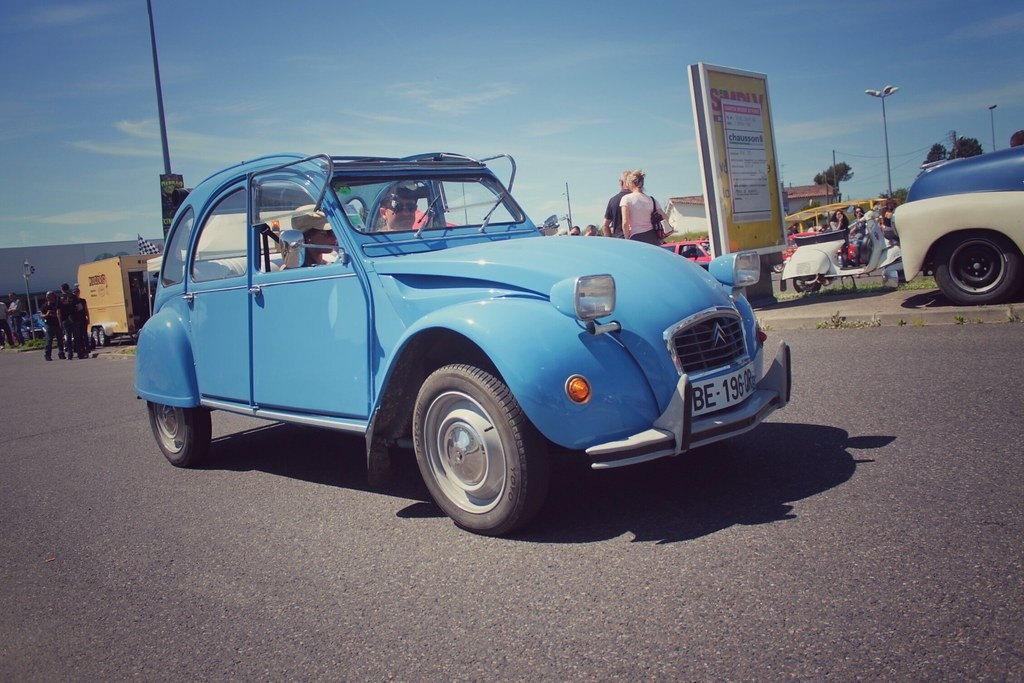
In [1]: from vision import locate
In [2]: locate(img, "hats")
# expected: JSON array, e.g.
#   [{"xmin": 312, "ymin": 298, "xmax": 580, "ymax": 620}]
[
  {"xmin": 291, "ymin": 203, "xmax": 332, "ymax": 234},
  {"xmin": 381, "ymin": 184, "xmax": 419, "ymax": 207}
]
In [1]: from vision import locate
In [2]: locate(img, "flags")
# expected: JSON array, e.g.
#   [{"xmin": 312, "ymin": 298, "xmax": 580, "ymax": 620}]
[{"xmin": 137, "ymin": 234, "xmax": 161, "ymax": 254}]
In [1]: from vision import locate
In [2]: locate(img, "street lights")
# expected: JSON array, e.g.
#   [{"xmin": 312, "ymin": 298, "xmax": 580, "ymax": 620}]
[
  {"xmin": 988, "ymin": 105, "xmax": 997, "ymax": 150},
  {"xmin": 866, "ymin": 84, "xmax": 901, "ymax": 199},
  {"xmin": 24, "ymin": 260, "xmax": 36, "ymax": 340}
]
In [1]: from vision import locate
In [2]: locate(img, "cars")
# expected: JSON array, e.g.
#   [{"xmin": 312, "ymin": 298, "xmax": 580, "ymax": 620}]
[
  {"xmin": 772, "ymin": 231, "xmax": 825, "ymax": 274},
  {"xmin": 892, "ymin": 131, "xmax": 1024, "ymax": 307},
  {"xmin": 134, "ymin": 151, "xmax": 792, "ymax": 539},
  {"xmin": 1, "ymin": 310, "xmax": 65, "ymax": 344},
  {"xmin": 660, "ymin": 240, "xmax": 712, "ymax": 269}
]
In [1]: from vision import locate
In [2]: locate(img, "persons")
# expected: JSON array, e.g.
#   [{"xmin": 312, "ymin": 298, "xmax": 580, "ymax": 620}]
[
  {"xmin": 620, "ymin": 169, "xmax": 669, "ymax": 246},
  {"xmin": 570, "ymin": 226, "xmax": 580, "ymax": 235},
  {"xmin": 882, "ymin": 199, "xmax": 898, "ymax": 240},
  {"xmin": 786, "ymin": 223, "xmax": 832, "ymax": 235},
  {"xmin": 39, "ymin": 284, "xmax": 91, "ymax": 360},
  {"xmin": 0, "ymin": 301, "xmax": 17, "ymax": 349},
  {"xmin": 850, "ymin": 206, "xmax": 873, "ymax": 278},
  {"xmin": 583, "ymin": 225, "xmax": 597, "ymax": 236},
  {"xmin": 600, "ymin": 170, "xmax": 632, "ymax": 239},
  {"xmin": 7, "ymin": 291, "xmax": 26, "ymax": 347},
  {"xmin": 829, "ymin": 210, "xmax": 852, "ymax": 279},
  {"xmin": 372, "ymin": 181, "xmax": 420, "ymax": 230},
  {"xmin": 273, "ymin": 203, "xmax": 338, "ymax": 270}
]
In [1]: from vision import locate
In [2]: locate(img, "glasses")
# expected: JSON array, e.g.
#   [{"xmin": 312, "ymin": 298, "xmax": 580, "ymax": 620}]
[
  {"xmin": 856, "ymin": 211, "xmax": 862, "ymax": 214},
  {"xmin": 73, "ymin": 291, "xmax": 80, "ymax": 293},
  {"xmin": 384, "ymin": 203, "xmax": 418, "ymax": 212},
  {"xmin": 570, "ymin": 233, "xmax": 579, "ymax": 235}
]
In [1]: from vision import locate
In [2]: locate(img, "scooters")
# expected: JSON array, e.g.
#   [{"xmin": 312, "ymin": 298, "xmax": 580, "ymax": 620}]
[{"xmin": 782, "ymin": 196, "xmax": 906, "ymax": 297}]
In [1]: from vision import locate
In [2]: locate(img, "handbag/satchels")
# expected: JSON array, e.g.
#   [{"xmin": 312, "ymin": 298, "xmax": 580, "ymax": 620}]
[{"xmin": 650, "ymin": 195, "xmax": 674, "ymax": 239}]
